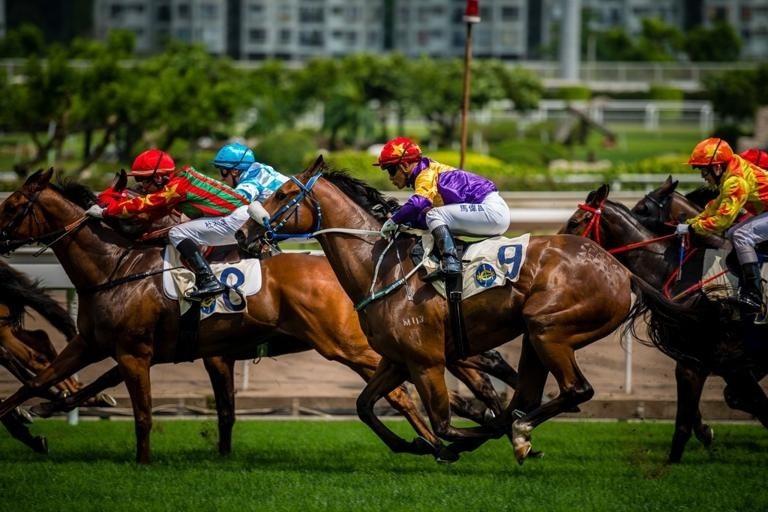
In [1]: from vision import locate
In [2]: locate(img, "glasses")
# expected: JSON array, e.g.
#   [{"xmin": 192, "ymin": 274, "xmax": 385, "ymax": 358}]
[
  {"xmin": 135, "ymin": 151, "xmax": 163, "ymax": 182},
  {"xmin": 386, "ymin": 142, "xmax": 413, "ymax": 177},
  {"xmin": 701, "ymin": 139, "xmax": 723, "ymax": 177},
  {"xmin": 220, "ymin": 148, "xmax": 249, "ymax": 177}
]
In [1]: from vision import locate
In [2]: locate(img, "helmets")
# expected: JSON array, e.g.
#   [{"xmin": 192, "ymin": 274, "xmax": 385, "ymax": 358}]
[
  {"xmin": 371, "ymin": 138, "xmax": 422, "ymax": 169},
  {"xmin": 126, "ymin": 150, "xmax": 177, "ymax": 177},
  {"xmin": 687, "ymin": 137, "xmax": 734, "ymax": 166},
  {"xmin": 210, "ymin": 143, "xmax": 256, "ymax": 172}
]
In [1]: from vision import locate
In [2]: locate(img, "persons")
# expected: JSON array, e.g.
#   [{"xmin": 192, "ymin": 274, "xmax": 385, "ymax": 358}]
[
  {"xmin": 675, "ymin": 138, "xmax": 768, "ymax": 309},
  {"xmin": 207, "ymin": 145, "xmax": 297, "ymax": 207},
  {"xmin": 369, "ymin": 140, "xmax": 510, "ymax": 281},
  {"xmin": 84, "ymin": 151, "xmax": 252, "ymax": 294}
]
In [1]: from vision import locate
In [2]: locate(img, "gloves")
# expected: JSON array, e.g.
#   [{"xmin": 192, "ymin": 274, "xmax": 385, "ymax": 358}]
[
  {"xmin": 380, "ymin": 218, "xmax": 397, "ymax": 243},
  {"xmin": 675, "ymin": 224, "xmax": 694, "ymax": 237},
  {"xmin": 85, "ymin": 204, "xmax": 105, "ymax": 221}
]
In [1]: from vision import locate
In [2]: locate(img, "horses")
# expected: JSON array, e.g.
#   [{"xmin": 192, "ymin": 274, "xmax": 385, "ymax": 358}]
[
  {"xmin": 233, "ymin": 154, "xmax": 738, "ymax": 467},
  {"xmin": 29, "ymin": 168, "xmax": 236, "ymax": 457},
  {"xmin": 0, "ymin": 256, "xmax": 117, "ymax": 408},
  {"xmin": 632, "ymin": 175, "xmax": 739, "ymax": 250},
  {"xmin": 555, "ymin": 183, "xmax": 768, "ymax": 465},
  {"xmin": 0, "ymin": 164, "xmax": 460, "ymax": 465}
]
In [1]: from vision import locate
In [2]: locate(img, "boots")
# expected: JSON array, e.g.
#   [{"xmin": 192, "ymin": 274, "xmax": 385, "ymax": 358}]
[
  {"xmin": 420, "ymin": 224, "xmax": 463, "ymax": 282},
  {"xmin": 737, "ymin": 263, "xmax": 762, "ymax": 312},
  {"xmin": 185, "ymin": 251, "xmax": 226, "ymax": 297}
]
